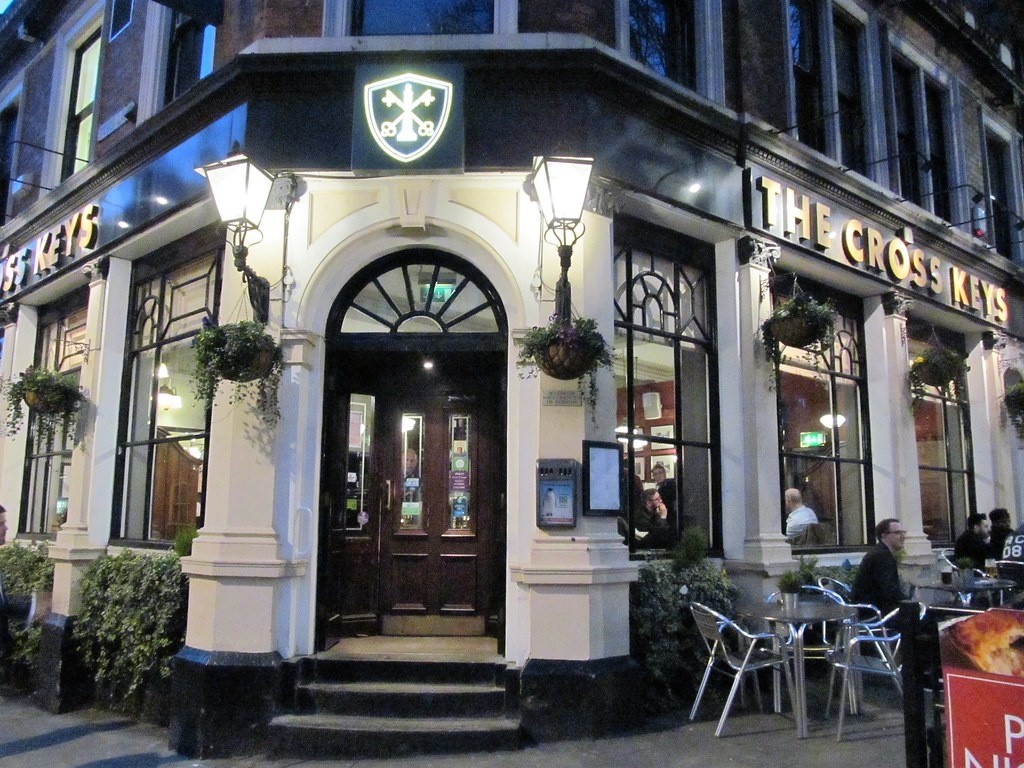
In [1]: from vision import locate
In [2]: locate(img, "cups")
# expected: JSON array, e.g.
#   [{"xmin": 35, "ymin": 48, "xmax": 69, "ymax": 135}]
[
  {"xmin": 941, "ymin": 566, "xmax": 952, "ymax": 586},
  {"xmin": 990, "ymin": 564, "xmax": 999, "ymax": 582},
  {"xmin": 985, "ymin": 559, "xmax": 995, "ymax": 579}
]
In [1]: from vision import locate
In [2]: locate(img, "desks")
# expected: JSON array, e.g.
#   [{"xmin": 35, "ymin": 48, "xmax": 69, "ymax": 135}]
[
  {"xmin": 910, "ymin": 577, "xmax": 1018, "ymax": 620},
  {"xmin": 744, "ymin": 597, "xmax": 859, "ymax": 742}
]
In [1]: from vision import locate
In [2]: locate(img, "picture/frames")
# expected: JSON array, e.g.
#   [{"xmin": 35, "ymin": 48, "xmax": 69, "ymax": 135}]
[{"xmin": 584, "ymin": 440, "xmax": 622, "ymax": 515}]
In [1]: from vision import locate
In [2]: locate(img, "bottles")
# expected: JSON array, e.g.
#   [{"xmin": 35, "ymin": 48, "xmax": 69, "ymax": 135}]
[
  {"xmin": 449, "ymin": 516, "xmax": 470, "ymax": 530},
  {"xmin": 452, "ymin": 447, "xmax": 468, "ymax": 472},
  {"xmin": 401, "ymin": 515, "xmax": 418, "ymax": 526}
]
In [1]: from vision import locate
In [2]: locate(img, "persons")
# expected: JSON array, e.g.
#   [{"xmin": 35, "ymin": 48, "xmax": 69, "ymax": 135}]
[
  {"xmin": 955, "ymin": 508, "xmax": 1024, "ymax": 592},
  {"xmin": 407, "ymin": 448, "xmax": 418, "ymax": 478},
  {"xmin": 0, "ymin": 505, "xmax": 37, "ymax": 665},
  {"xmin": 623, "ymin": 460, "xmax": 677, "ymax": 532},
  {"xmin": 785, "ymin": 489, "xmax": 818, "ymax": 537},
  {"xmin": 851, "ymin": 519, "xmax": 943, "ymax": 693}
]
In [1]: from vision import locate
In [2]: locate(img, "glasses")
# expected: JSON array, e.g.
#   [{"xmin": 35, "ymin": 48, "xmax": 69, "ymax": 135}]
[
  {"xmin": 879, "ymin": 530, "xmax": 906, "ymax": 537},
  {"xmin": 649, "ymin": 496, "xmax": 662, "ymax": 502}
]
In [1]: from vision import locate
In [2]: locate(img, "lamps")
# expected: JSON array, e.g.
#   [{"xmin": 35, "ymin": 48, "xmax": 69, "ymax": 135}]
[
  {"xmin": 523, "ymin": 134, "xmax": 594, "ymax": 309},
  {"xmin": 843, "ymin": 151, "xmax": 933, "ymax": 177},
  {"xmin": 774, "ymin": 101, "xmax": 861, "ymax": 137},
  {"xmin": 898, "ymin": 183, "xmax": 984, "ymax": 209},
  {"xmin": 194, "ymin": 143, "xmax": 275, "ymax": 274},
  {"xmin": 947, "ymin": 210, "xmax": 1024, "ymax": 237}
]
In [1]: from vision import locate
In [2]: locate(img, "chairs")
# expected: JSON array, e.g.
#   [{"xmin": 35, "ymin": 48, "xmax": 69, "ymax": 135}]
[
  {"xmin": 940, "ymin": 548, "xmax": 978, "ymax": 578},
  {"xmin": 995, "ymin": 560, "xmax": 1024, "ymax": 584},
  {"xmin": 766, "ymin": 586, "xmax": 856, "ymax": 715},
  {"xmin": 818, "ymin": 576, "xmax": 903, "ymax": 699},
  {"xmin": 826, "ymin": 600, "xmax": 927, "ymax": 743},
  {"xmin": 688, "ymin": 600, "xmax": 800, "ymax": 737}
]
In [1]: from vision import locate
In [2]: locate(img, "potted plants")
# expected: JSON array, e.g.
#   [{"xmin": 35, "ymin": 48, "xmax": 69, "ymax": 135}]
[
  {"xmin": 911, "ymin": 325, "xmax": 974, "ymax": 446},
  {"xmin": 758, "ymin": 272, "xmax": 839, "ymax": 391},
  {"xmin": 951, "ymin": 557, "xmax": 974, "ymax": 585},
  {"xmin": 517, "ymin": 311, "xmax": 618, "ymax": 426},
  {"xmin": 776, "ymin": 572, "xmax": 804, "ymax": 612},
  {"xmin": 7, "ymin": 367, "xmax": 84, "ymax": 434},
  {"xmin": 193, "ymin": 320, "xmax": 281, "ymax": 413}
]
